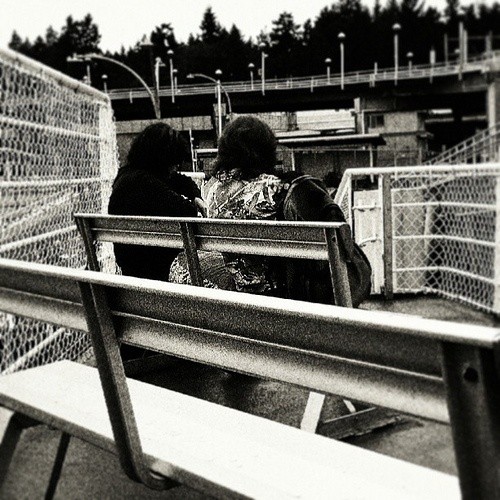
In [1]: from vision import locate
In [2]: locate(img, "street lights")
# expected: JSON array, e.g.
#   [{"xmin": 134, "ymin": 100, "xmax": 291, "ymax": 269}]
[
  {"xmin": 187, "ymin": 73, "xmax": 232, "ymax": 123},
  {"xmin": 173, "ymin": 68, "xmax": 177, "ymax": 90},
  {"xmin": 168, "ymin": 50, "xmax": 174, "ymax": 103},
  {"xmin": 85, "ymin": 57, "xmax": 91, "ymax": 87},
  {"xmin": 406, "ymin": 52, "xmax": 413, "ymax": 77},
  {"xmin": 325, "ymin": 58, "xmax": 331, "ymax": 84},
  {"xmin": 260, "ymin": 42, "xmax": 265, "ymax": 95},
  {"xmin": 102, "ymin": 75, "xmax": 107, "ymax": 93},
  {"xmin": 338, "ymin": 32, "xmax": 346, "ymax": 90},
  {"xmin": 392, "ymin": 23, "xmax": 401, "ymax": 85},
  {"xmin": 457, "ymin": 10, "xmax": 465, "ymax": 80},
  {"xmin": 248, "ymin": 63, "xmax": 254, "ymax": 90},
  {"xmin": 83, "ymin": 54, "xmax": 160, "ymax": 120}
]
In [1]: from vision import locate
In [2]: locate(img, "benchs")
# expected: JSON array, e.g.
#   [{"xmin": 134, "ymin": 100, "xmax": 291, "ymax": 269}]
[{"xmin": 0, "ymin": 213, "xmax": 500, "ymax": 500}]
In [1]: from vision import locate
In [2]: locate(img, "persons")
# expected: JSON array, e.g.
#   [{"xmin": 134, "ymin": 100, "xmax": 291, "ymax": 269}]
[
  {"xmin": 107, "ymin": 123, "xmax": 201, "ymax": 282},
  {"xmin": 201, "ymin": 115, "xmax": 290, "ymax": 291}
]
